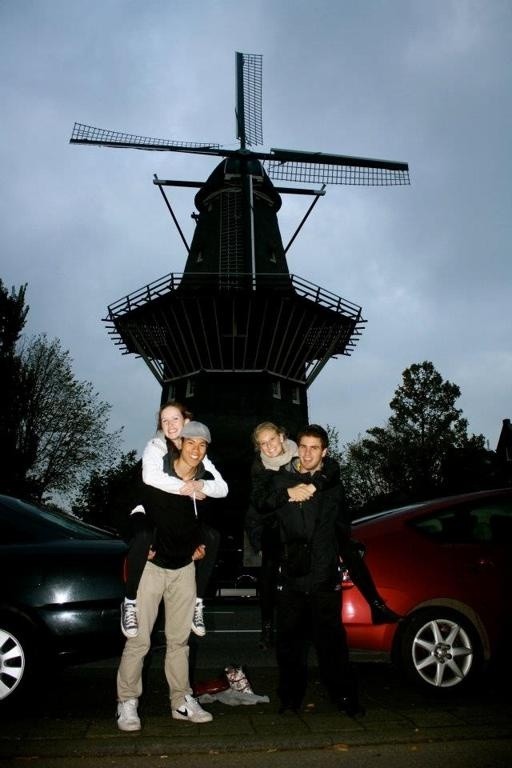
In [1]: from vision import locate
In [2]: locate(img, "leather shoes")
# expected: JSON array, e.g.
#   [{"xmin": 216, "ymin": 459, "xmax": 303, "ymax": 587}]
[{"xmin": 371, "ymin": 599, "xmax": 415, "ymax": 624}]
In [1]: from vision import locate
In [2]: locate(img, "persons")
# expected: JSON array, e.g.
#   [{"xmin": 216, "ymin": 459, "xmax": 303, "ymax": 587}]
[
  {"xmin": 260, "ymin": 422, "xmax": 365, "ymax": 719},
  {"xmin": 110, "ymin": 421, "xmax": 214, "ymax": 733},
  {"xmin": 242, "ymin": 420, "xmax": 404, "ymax": 656},
  {"xmin": 114, "ymin": 403, "xmax": 229, "ymax": 637}
]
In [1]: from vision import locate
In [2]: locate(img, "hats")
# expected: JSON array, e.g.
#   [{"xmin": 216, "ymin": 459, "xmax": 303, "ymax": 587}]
[{"xmin": 181, "ymin": 420, "xmax": 212, "ymax": 444}]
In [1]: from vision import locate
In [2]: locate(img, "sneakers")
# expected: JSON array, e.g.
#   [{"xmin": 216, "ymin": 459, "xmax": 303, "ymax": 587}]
[
  {"xmin": 191, "ymin": 602, "xmax": 206, "ymax": 637},
  {"xmin": 171, "ymin": 695, "xmax": 213, "ymax": 723},
  {"xmin": 120, "ymin": 601, "xmax": 138, "ymax": 638},
  {"xmin": 116, "ymin": 702, "xmax": 142, "ymax": 731}
]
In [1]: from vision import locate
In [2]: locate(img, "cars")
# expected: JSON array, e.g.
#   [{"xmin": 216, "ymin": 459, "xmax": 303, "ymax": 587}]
[
  {"xmin": 336, "ymin": 487, "xmax": 511, "ymax": 699},
  {"xmin": 0, "ymin": 491, "xmax": 130, "ymax": 709}
]
